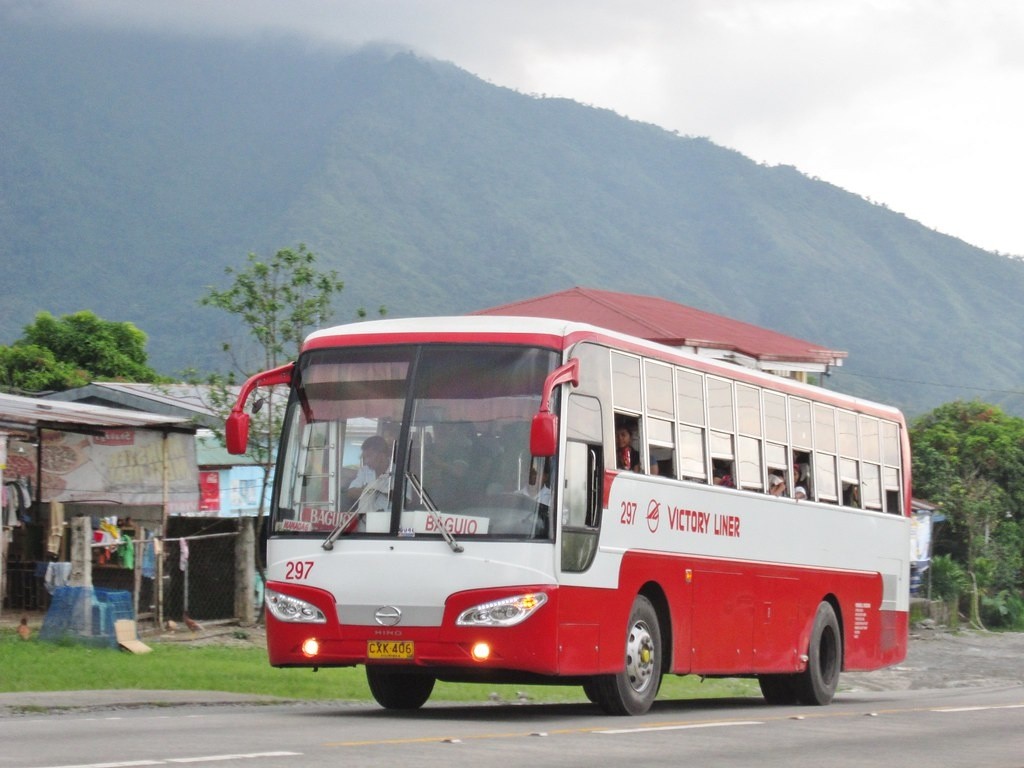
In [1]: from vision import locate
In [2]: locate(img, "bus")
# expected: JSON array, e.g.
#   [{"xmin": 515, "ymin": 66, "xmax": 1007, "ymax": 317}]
[{"xmin": 223, "ymin": 315, "xmax": 914, "ymax": 717}]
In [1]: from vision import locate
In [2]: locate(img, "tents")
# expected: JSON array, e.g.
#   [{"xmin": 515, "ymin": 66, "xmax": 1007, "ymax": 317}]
[{"xmin": 0, "ymin": 392, "xmax": 192, "ymax": 632}]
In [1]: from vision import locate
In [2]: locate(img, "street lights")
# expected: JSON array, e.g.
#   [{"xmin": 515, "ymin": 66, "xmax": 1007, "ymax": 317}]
[{"xmin": 981, "ymin": 510, "xmax": 1014, "ymax": 555}]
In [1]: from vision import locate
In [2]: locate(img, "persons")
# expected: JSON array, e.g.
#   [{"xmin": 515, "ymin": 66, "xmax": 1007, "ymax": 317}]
[
  {"xmin": 346, "ymin": 436, "xmax": 412, "ymax": 514},
  {"xmin": 712, "ymin": 460, "xmax": 734, "ymax": 486},
  {"xmin": 616, "ymin": 424, "xmax": 639, "ymax": 473},
  {"xmin": 842, "ymin": 485, "xmax": 859, "ymax": 507},
  {"xmin": 768, "ymin": 450, "xmax": 810, "ymax": 500},
  {"xmin": 650, "ymin": 454, "xmax": 659, "ymax": 474},
  {"xmin": 379, "ymin": 419, "xmax": 552, "ymax": 509}
]
